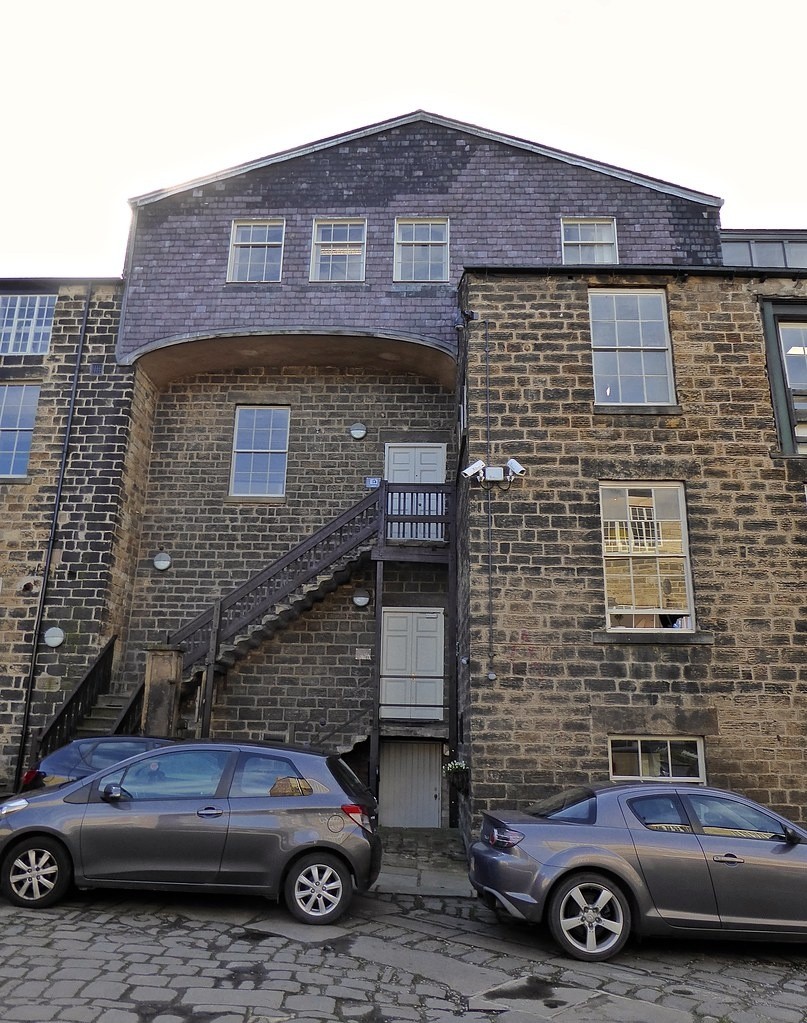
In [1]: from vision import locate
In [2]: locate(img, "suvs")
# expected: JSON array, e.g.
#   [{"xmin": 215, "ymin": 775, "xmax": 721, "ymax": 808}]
[{"xmin": 19, "ymin": 734, "xmax": 202, "ymax": 792}]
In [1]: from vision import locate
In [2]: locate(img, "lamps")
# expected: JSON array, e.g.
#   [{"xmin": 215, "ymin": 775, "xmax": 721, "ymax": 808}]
[{"xmin": 461, "ymin": 459, "xmax": 526, "ymax": 491}]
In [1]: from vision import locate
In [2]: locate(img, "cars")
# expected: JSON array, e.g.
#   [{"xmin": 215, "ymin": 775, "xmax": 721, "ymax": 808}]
[
  {"xmin": 468, "ymin": 780, "xmax": 807, "ymax": 962},
  {"xmin": 0, "ymin": 739, "xmax": 385, "ymax": 925}
]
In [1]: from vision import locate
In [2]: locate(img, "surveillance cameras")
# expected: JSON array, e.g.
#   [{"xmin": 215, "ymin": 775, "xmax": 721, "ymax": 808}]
[
  {"xmin": 507, "ymin": 459, "xmax": 526, "ymax": 476},
  {"xmin": 461, "ymin": 460, "xmax": 486, "ymax": 478}
]
